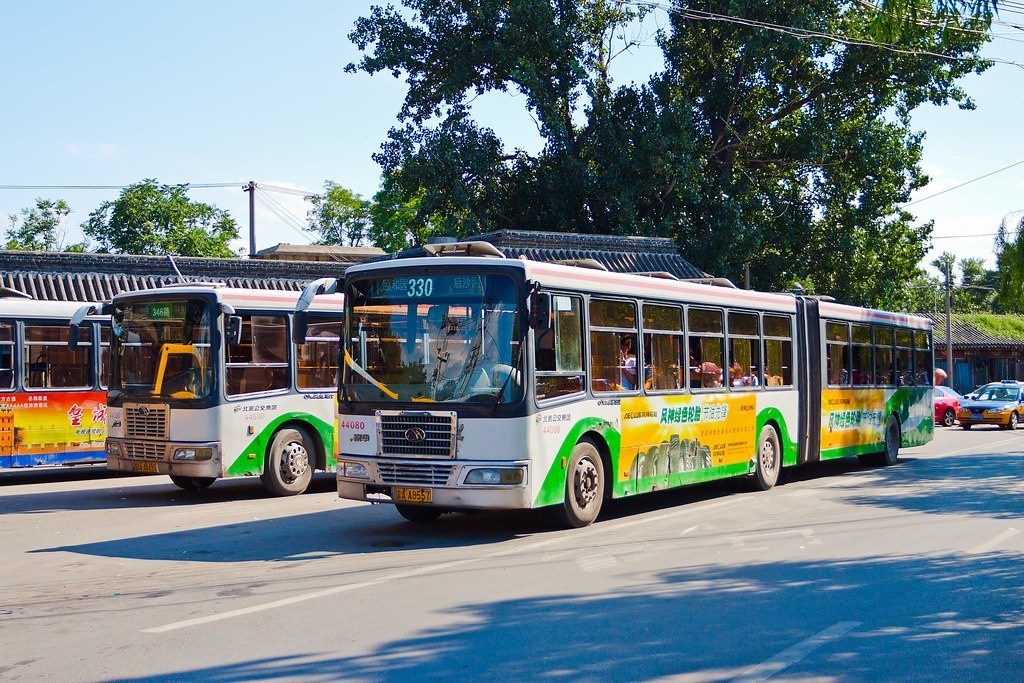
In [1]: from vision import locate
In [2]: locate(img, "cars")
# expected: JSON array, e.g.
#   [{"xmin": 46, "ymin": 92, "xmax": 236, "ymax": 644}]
[{"xmin": 935, "ymin": 379, "xmax": 1024, "ymax": 431}]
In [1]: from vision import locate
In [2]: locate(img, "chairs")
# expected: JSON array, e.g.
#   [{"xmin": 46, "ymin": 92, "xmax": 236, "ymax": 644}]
[
  {"xmin": 191, "ymin": 352, "xmax": 421, "ymax": 395},
  {"xmin": 0, "ymin": 353, "xmax": 154, "ymax": 389}
]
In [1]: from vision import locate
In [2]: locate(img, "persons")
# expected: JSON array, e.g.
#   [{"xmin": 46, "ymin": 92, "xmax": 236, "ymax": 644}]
[
  {"xmin": 590, "ymin": 331, "xmax": 932, "ymax": 391},
  {"xmin": 239, "ymin": 350, "xmax": 274, "ymax": 394}
]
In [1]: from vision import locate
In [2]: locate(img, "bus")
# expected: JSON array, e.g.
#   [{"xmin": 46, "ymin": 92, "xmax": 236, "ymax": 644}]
[
  {"xmin": 1, "ymin": 294, "xmax": 184, "ymax": 472},
  {"xmin": 293, "ymin": 240, "xmax": 935, "ymax": 531},
  {"xmin": 68, "ymin": 282, "xmax": 473, "ymax": 496}
]
